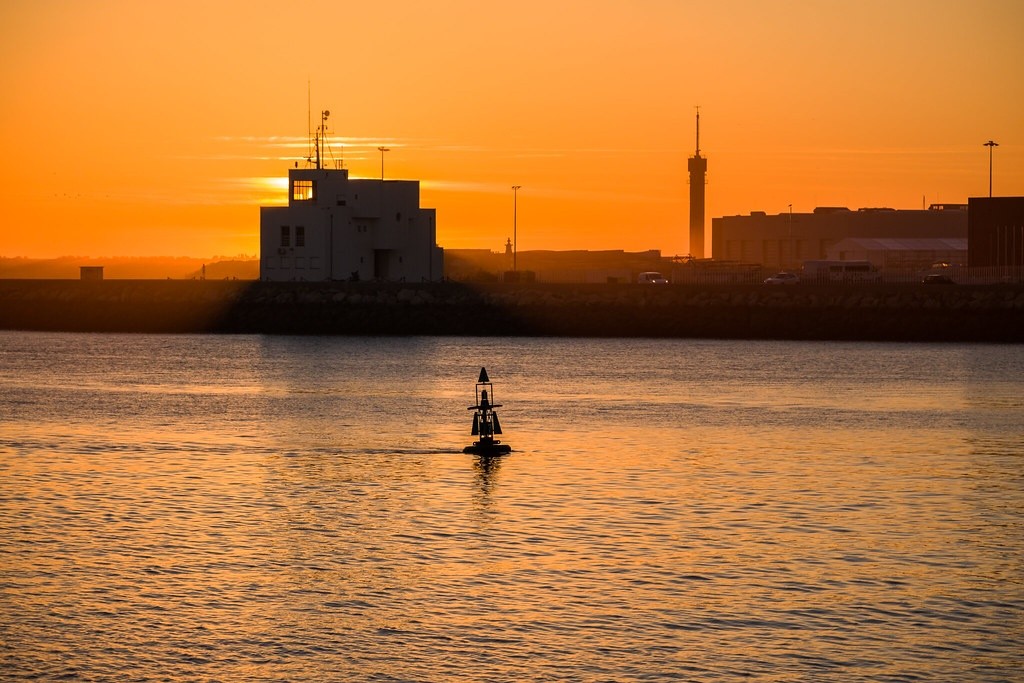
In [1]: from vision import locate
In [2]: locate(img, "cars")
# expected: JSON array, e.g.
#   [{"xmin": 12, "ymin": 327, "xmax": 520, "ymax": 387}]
[
  {"xmin": 638, "ymin": 272, "xmax": 668, "ymax": 285},
  {"xmin": 765, "ymin": 271, "xmax": 800, "ymax": 285},
  {"xmin": 922, "ymin": 274, "xmax": 956, "ymax": 284}
]
